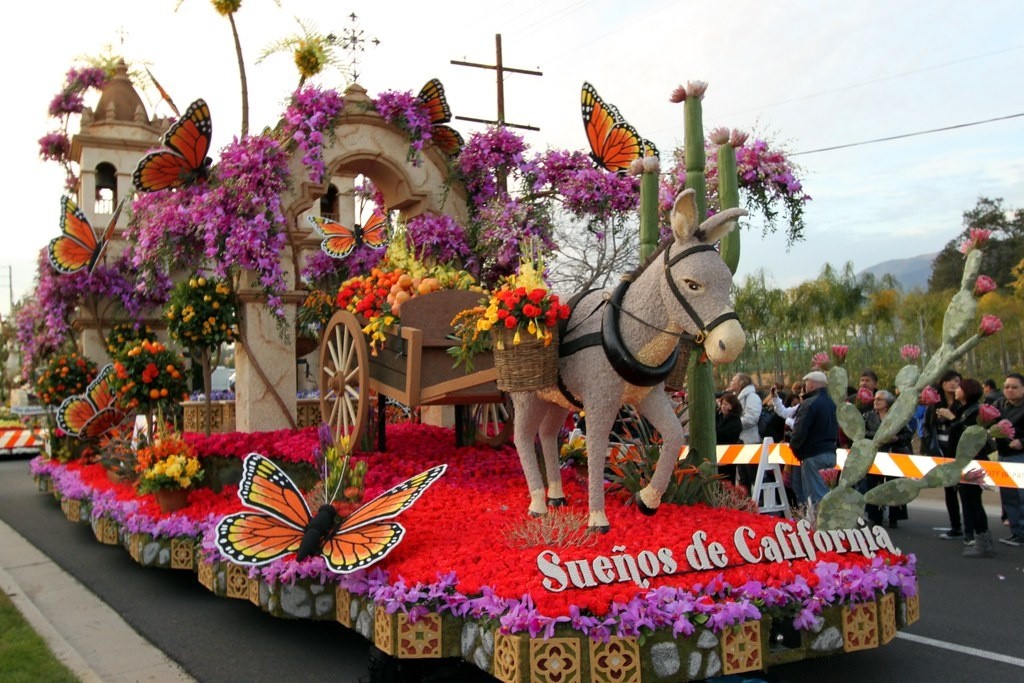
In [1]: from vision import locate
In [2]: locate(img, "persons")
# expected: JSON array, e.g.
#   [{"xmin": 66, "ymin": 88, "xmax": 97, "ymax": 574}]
[
  {"xmin": 577, "ymin": 411, "xmax": 586, "ymax": 437},
  {"xmin": 789, "ymin": 373, "xmax": 839, "ymax": 518},
  {"xmin": 715, "ymin": 371, "xmax": 1024, "ymax": 559},
  {"xmin": 674, "ymin": 392, "xmax": 686, "ymax": 415}
]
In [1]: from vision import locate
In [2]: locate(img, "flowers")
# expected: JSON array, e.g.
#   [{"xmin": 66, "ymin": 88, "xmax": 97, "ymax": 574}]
[
  {"xmin": 445, "ymin": 242, "xmax": 571, "ymax": 374},
  {"xmin": 141, "ymin": 457, "xmax": 203, "ymax": 493}
]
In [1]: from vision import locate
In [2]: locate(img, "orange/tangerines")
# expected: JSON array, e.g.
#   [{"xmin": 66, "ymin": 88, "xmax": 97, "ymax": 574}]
[
  {"xmin": 358, "ymin": 267, "xmax": 402, "ymax": 294},
  {"xmin": 38, "ymin": 277, "xmax": 232, "ymax": 420}
]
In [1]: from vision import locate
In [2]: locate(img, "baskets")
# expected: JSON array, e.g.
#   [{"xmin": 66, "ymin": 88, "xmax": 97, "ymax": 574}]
[{"xmin": 490, "ymin": 317, "xmax": 559, "ymax": 391}]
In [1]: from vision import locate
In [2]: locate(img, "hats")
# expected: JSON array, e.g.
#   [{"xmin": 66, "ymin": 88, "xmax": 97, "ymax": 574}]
[{"xmin": 803, "ymin": 371, "xmax": 826, "ymax": 382}]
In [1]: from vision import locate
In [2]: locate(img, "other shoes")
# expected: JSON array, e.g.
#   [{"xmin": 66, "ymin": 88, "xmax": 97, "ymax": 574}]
[
  {"xmin": 998, "ymin": 533, "xmax": 1024, "ymax": 546},
  {"xmin": 940, "ymin": 533, "xmax": 964, "ymax": 539},
  {"xmin": 889, "ymin": 519, "xmax": 898, "ymax": 529},
  {"xmin": 963, "ymin": 536, "xmax": 976, "ymax": 545},
  {"xmin": 1003, "ymin": 518, "xmax": 1009, "ymax": 526}
]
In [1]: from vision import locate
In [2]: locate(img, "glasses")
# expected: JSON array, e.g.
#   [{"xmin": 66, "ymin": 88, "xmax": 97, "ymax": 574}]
[{"xmin": 873, "ymin": 397, "xmax": 885, "ymax": 401}]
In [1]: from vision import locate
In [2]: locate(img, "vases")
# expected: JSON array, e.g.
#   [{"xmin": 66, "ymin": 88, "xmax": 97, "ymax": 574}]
[
  {"xmin": 488, "ymin": 315, "xmax": 559, "ymax": 392},
  {"xmin": 158, "ymin": 486, "xmax": 190, "ymax": 513}
]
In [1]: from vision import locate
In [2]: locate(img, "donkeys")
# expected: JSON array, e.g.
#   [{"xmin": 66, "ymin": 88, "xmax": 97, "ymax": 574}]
[{"xmin": 506, "ymin": 186, "xmax": 749, "ymax": 532}]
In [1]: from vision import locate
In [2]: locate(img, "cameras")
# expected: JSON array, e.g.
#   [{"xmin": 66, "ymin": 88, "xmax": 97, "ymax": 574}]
[
  {"xmin": 715, "ymin": 390, "xmax": 731, "ymax": 398},
  {"xmin": 772, "ymin": 388, "xmax": 775, "ymax": 393}
]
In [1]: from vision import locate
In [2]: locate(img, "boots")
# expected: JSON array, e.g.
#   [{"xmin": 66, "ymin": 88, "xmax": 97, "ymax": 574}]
[{"xmin": 962, "ymin": 529, "xmax": 997, "ymax": 557}]
